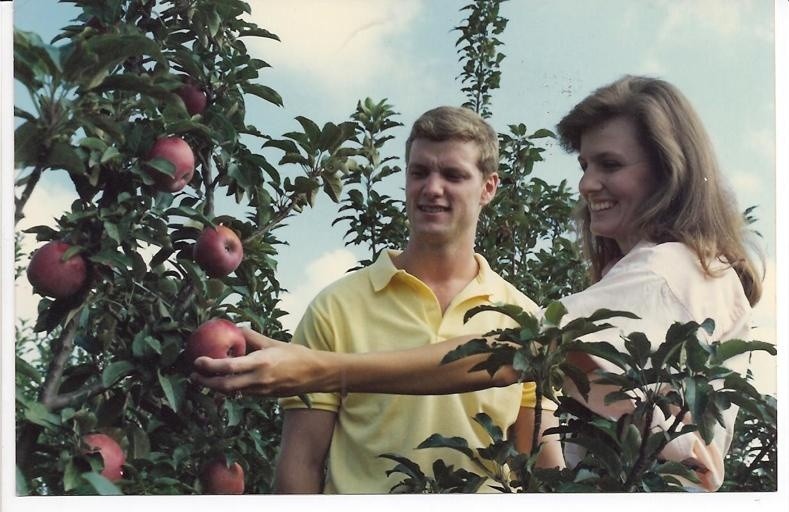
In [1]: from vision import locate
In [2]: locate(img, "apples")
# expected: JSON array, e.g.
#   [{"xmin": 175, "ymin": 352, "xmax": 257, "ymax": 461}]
[
  {"xmin": 193, "ymin": 225, "xmax": 243, "ymax": 278},
  {"xmin": 80, "ymin": 434, "xmax": 126, "ymax": 481},
  {"xmin": 142, "ymin": 137, "xmax": 194, "ymax": 193},
  {"xmin": 175, "ymin": 84, "xmax": 207, "ymax": 117},
  {"xmin": 27, "ymin": 241, "xmax": 86, "ymax": 300},
  {"xmin": 200, "ymin": 461, "xmax": 244, "ymax": 495},
  {"xmin": 185, "ymin": 318, "xmax": 246, "ymax": 378}
]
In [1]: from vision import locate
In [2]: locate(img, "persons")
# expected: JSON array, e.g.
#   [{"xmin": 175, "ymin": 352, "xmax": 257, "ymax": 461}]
[
  {"xmin": 183, "ymin": 69, "xmax": 767, "ymax": 493},
  {"xmin": 266, "ymin": 103, "xmax": 573, "ymax": 494}
]
